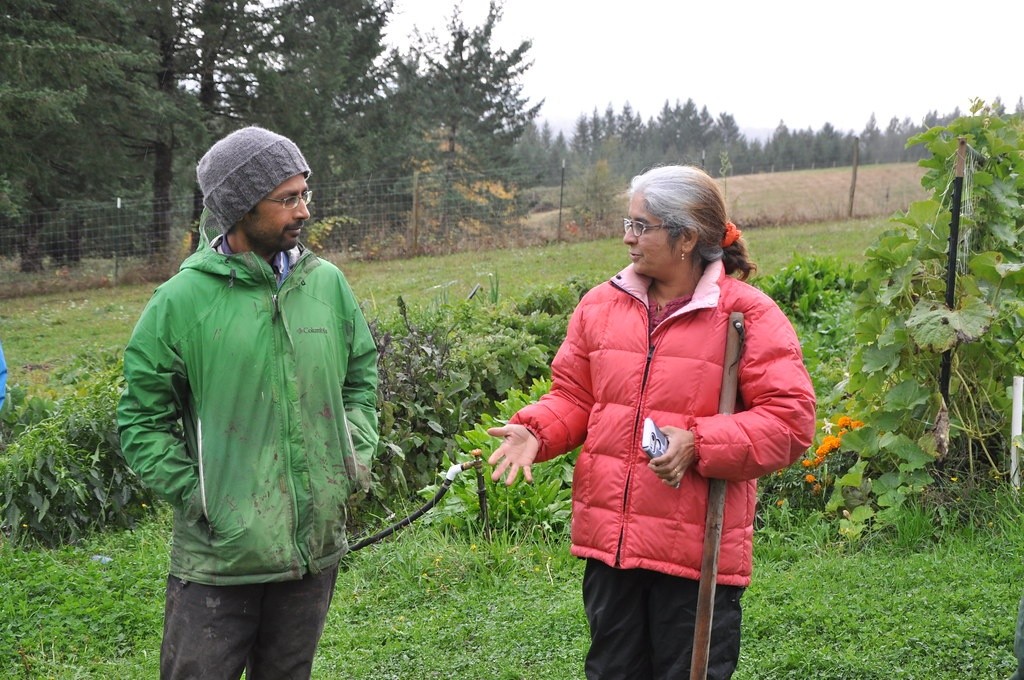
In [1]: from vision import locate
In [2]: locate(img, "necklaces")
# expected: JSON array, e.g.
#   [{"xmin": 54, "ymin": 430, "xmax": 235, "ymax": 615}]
[{"xmin": 652, "ymin": 285, "xmax": 687, "ymax": 317}]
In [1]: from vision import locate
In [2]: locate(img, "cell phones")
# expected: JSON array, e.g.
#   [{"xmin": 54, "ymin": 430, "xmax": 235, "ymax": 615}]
[{"xmin": 642, "ymin": 417, "xmax": 680, "ymax": 488}]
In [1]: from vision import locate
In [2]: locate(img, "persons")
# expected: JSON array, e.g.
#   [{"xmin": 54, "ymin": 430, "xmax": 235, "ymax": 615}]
[
  {"xmin": 115, "ymin": 123, "xmax": 381, "ymax": 680},
  {"xmin": 485, "ymin": 163, "xmax": 818, "ymax": 680}
]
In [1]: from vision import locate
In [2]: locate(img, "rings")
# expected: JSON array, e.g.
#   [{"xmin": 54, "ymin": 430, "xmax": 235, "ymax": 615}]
[{"xmin": 673, "ymin": 469, "xmax": 681, "ymax": 477}]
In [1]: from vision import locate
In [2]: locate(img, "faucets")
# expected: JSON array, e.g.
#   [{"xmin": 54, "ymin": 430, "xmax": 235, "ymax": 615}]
[{"xmin": 453, "ymin": 448, "xmax": 483, "ymax": 476}]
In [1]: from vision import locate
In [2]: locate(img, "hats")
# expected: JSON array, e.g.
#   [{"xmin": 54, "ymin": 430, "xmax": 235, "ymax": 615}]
[{"xmin": 196, "ymin": 125, "xmax": 311, "ymax": 235}]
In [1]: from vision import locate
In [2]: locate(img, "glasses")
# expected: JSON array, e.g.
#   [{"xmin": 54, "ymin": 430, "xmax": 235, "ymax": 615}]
[
  {"xmin": 623, "ymin": 218, "xmax": 672, "ymax": 237},
  {"xmin": 262, "ymin": 190, "xmax": 312, "ymax": 211}
]
